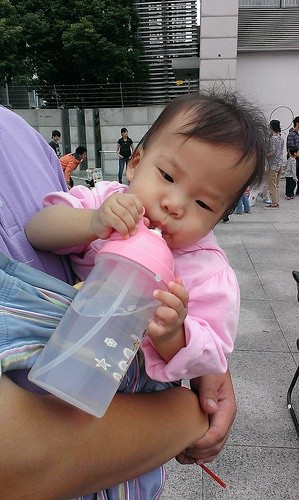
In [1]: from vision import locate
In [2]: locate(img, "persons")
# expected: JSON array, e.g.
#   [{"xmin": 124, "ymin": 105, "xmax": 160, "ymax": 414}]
[
  {"xmin": 283, "ymin": 146, "xmax": 299, "ymax": 199},
  {"xmin": 223, "ymin": 215, "xmax": 230, "ymax": 224},
  {"xmin": 287, "ymin": 116, "xmax": 299, "ymax": 196},
  {"xmin": 117, "ymin": 127, "xmax": 134, "ymax": 184},
  {"xmin": 235, "ymin": 191, "xmax": 250, "ymax": 214},
  {"xmin": 25, "ymin": 82, "xmax": 274, "ymax": 383},
  {"xmin": 48, "ymin": 130, "xmax": 62, "ymax": 159},
  {"xmin": 264, "ymin": 120, "xmax": 282, "ymax": 209},
  {"xmin": 259, "ymin": 134, "xmax": 282, "ymax": 203},
  {"xmin": 0, "ymin": 103, "xmax": 239, "ymax": 499},
  {"xmin": 59, "ymin": 146, "xmax": 87, "ymax": 190}
]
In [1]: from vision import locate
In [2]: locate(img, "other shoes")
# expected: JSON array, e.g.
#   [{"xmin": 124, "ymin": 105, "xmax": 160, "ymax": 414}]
[
  {"xmin": 223, "ymin": 220, "xmax": 229, "ymax": 224},
  {"xmin": 264, "ymin": 203, "xmax": 280, "ymax": 210},
  {"xmin": 286, "ymin": 196, "xmax": 294, "ymax": 200},
  {"xmin": 234, "ymin": 212, "xmax": 243, "ymax": 215},
  {"xmin": 243, "ymin": 210, "xmax": 252, "ymax": 213}
]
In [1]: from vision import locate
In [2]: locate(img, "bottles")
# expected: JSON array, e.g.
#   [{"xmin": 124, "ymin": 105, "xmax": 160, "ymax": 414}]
[{"xmin": 28, "ymin": 206, "xmax": 178, "ymax": 419}]
